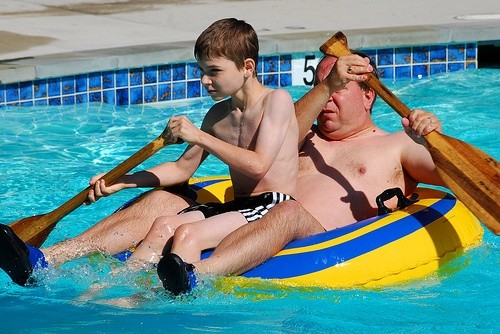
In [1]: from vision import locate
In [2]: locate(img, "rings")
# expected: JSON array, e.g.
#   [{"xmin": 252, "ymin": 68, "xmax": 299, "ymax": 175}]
[
  {"xmin": 348, "ymin": 66, "xmax": 351, "ymax": 73},
  {"xmin": 427, "ymin": 117, "xmax": 432, "ymax": 123}
]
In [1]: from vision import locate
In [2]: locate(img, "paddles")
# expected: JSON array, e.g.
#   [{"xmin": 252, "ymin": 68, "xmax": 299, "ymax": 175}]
[
  {"xmin": 318, "ymin": 28, "xmax": 500, "ymax": 240},
  {"xmin": 7, "ymin": 128, "xmax": 185, "ymax": 249}
]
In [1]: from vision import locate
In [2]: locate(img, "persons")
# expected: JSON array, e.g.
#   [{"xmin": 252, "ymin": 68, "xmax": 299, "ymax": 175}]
[
  {"xmin": 131, "ymin": 50, "xmax": 442, "ymax": 287},
  {"xmin": 0, "ymin": 17, "xmax": 298, "ymax": 287}
]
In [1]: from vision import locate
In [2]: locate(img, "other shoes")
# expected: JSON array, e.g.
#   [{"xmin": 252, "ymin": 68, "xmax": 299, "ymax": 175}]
[
  {"xmin": 157, "ymin": 253, "xmax": 198, "ymax": 302},
  {"xmin": 0, "ymin": 224, "xmax": 48, "ymax": 288}
]
